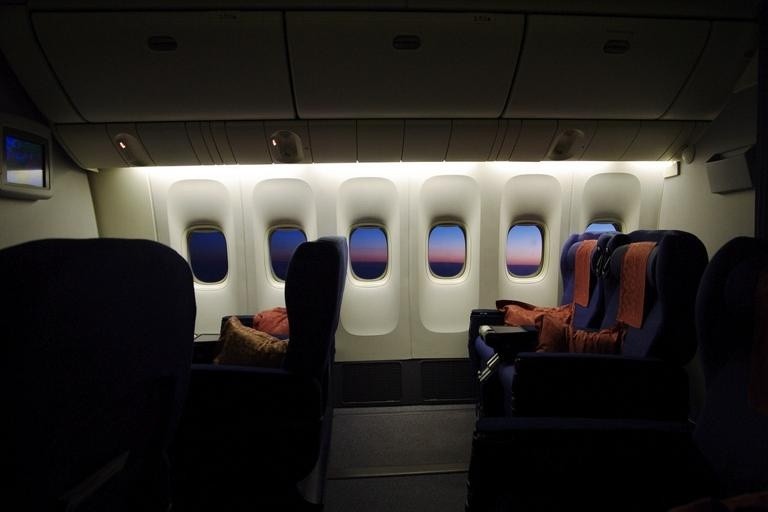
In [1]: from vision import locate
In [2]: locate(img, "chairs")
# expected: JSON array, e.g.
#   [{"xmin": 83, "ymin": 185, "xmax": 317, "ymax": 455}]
[
  {"xmin": 466, "ymin": 228, "xmax": 768, "ymax": 512},
  {"xmin": 190, "ymin": 237, "xmax": 349, "ymax": 511},
  {"xmin": 0, "ymin": 238, "xmax": 196, "ymax": 512}
]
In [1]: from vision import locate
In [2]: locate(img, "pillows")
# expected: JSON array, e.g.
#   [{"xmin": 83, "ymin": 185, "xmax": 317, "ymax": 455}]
[
  {"xmin": 251, "ymin": 308, "xmax": 290, "ymax": 338},
  {"xmin": 214, "ymin": 315, "xmax": 289, "ymax": 369}
]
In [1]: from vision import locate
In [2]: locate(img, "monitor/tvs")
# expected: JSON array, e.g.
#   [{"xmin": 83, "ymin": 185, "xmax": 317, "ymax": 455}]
[{"xmin": 0, "ymin": 111, "xmax": 55, "ymax": 200}]
